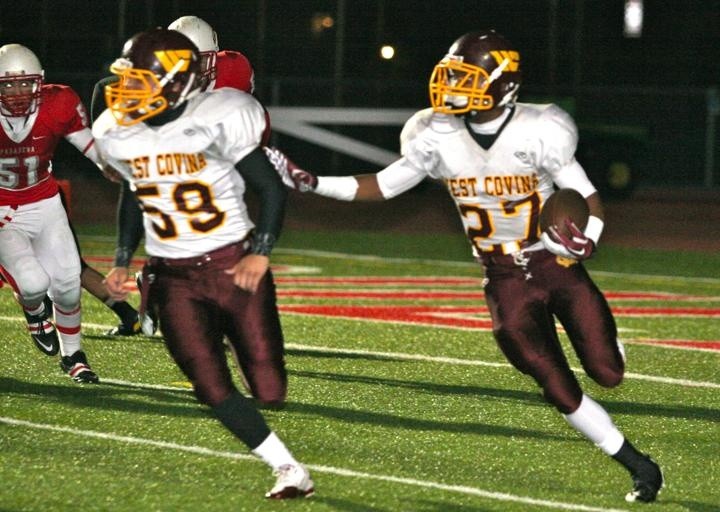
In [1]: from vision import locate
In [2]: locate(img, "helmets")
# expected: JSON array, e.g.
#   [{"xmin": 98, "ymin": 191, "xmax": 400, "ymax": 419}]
[
  {"xmin": 102, "ymin": 15, "xmax": 257, "ymax": 129},
  {"xmin": 428, "ymin": 29, "xmax": 523, "ymax": 121},
  {"xmin": 0, "ymin": 44, "xmax": 46, "ymax": 117}
]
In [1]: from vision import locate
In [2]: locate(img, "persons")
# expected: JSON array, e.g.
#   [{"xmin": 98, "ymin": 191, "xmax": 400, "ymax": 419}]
[
  {"xmin": 87, "ymin": 29, "xmax": 317, "ymax": 504},
  {"xmin": 48, "ymin": 170, "xmax": 144, "ymax": 339},
  {"xmin": 259, "ymin": 26, "xmax": 664, "ymax": 504},
  {"xmin": 86, "ymin": 14, "xmax": 264, "ymax": 345},
  {"xmin": 1, "ymin": 41, "xmax": 125, "ymax": 386}
]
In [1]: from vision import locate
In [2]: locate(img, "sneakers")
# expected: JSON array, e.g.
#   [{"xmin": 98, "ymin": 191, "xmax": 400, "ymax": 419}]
[
  {"xmin": 263, "ymin": 463, "xmax": 315, "ymax": 499},
  {"xmin": 22, "ymin": 302, "xmax": 59, "ymax": 357},
  {"xmin": 104, "ymin": 313, "xmax": 143, "ymax": 337},
  {"xmin": 136, "ymin": 271, "xmax": 159, "ymax": 337},
  {"xmin": 623, "ymin": 454, "xmax": 666, "ymax": 504},
  {"xmin": 59, "ymin": 349, "xmax": 99, "ymax": 384}
]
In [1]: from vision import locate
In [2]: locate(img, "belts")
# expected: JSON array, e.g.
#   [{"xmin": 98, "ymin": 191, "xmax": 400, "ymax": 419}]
[
  {"xmin": 150, "ymin": 239, "xmax": 252, "ymax": 268},
  {"xmin": 483, "ymin": 249, "xmax": 551, "ymax": 265}
]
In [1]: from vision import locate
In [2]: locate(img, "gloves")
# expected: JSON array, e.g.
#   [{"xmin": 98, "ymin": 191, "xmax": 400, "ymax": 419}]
[
  {"xmin": 538, "ymin": 216, "xmax": 596, "ymax": 262},
  {"xmin": 262, "ymin": 144, "xmax": 317, "ymax": 195}
]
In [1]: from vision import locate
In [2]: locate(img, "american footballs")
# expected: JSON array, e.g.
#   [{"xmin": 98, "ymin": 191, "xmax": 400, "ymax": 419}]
[{"xmin": 540, "ymin": 189, "xmax": 589, "ymax": 243}]
